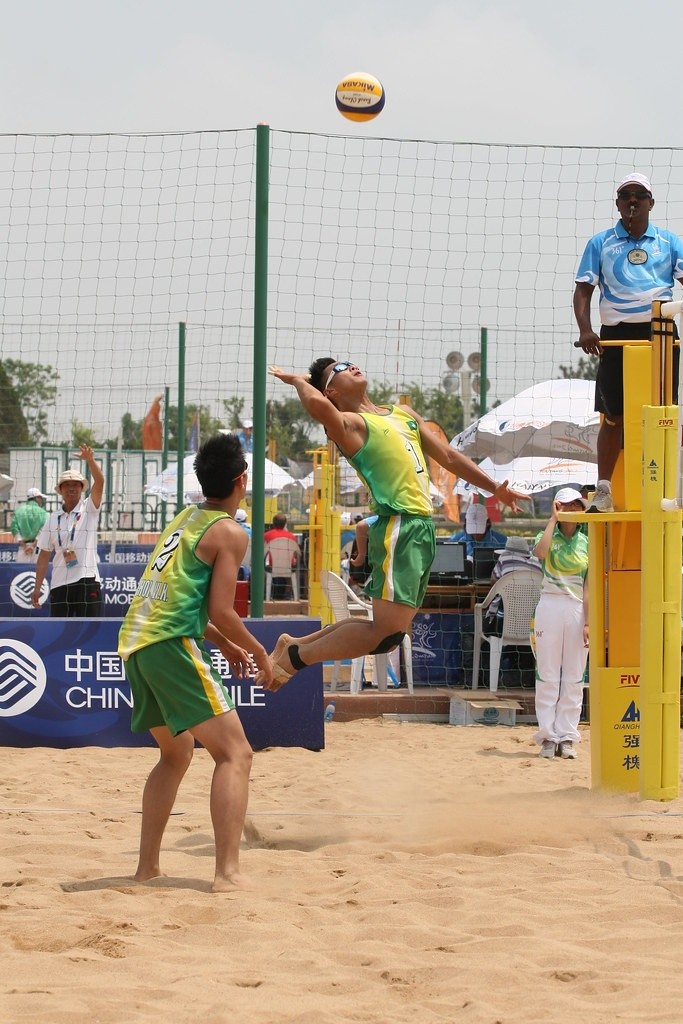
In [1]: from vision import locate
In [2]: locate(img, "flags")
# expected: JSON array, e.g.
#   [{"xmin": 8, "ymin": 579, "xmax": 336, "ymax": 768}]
[
  {"xmin": 422, "ymin": 419, "xmax": 460, "ymax": 524},
  {"xmin": 142, "ymin": 394, "xmax": 163, "ymax": 450},
  {"xmin": 186, "ymin": 410, "xmax": 199, "ymax": 452}
]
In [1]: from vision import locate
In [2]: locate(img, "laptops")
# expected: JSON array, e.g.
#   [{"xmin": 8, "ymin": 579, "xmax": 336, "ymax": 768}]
[{"xmin": 425, "ymin": 541, "xmax": 469, "ymax": 581}]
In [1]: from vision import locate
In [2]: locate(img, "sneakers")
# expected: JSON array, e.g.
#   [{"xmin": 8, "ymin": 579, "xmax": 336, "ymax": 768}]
[
  {"xmin": 584, "ymin": 484, "xmax": 615, "ymax": 514},
  {"xmin": 558, "ymin": 742, "xmax": 577, "ymax": 759},
  {"xmin": 538, "ymin": 740, "xmax": 557, "ymax": 760}
]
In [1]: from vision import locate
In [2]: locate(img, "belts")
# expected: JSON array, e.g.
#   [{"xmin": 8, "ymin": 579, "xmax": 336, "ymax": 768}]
[{"xmin": 24, "ymin": 539, "xmax": 35, "ymax": 543}]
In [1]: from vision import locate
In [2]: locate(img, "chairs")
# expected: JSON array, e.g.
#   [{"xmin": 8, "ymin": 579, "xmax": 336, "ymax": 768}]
[
  {"xmin": 239, "ymin": 534, "xmax": 251, "ymax": 582},
  {"xmin": 264, "ymin": 537, "xmax": 301, "ymax": 602},
  {"xmin": 319, "ymin": 570, "xmax": 414, "ymax": 693},
  {"xmin": 472, "ymin": 568, "xmax": 544, "ymax": 692}
]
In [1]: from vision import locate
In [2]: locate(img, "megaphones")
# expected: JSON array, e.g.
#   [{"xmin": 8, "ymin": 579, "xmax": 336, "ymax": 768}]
[
  {"xmin": 447, "ymin": 351, "xmax": 464, "ymax": 369},
  {"xmin": 472, "ymin": 378, "xmax": 491, "ymax": 393},
  {"xmin": 443, "ymin": 375, "xmax": 459, "ymax": 391},
  {"xmin": 468, "ymin": 352, "xmax": 481, "ymax": 370}
]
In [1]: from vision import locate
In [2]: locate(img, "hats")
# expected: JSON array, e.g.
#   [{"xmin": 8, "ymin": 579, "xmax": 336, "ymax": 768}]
[
  {"xmin": 26, "ymin": 487, "xmax": 48, "ymax": 500},
  {"xmin": 616, "ymin": 173, "xmax": 652, "ymax": 194},
  {"xmin": 465, "ymin": 503, "xmax": 488, "ymax": 534},
  {"xmin": 341, "ymin": 512, "xmax": 352, "ymax": 526},
  {"xmin": 234, "ymin": 509, "xmax": 249, "ymax": 521},
  {"xmin": 494, "ymin": 537, "xmax": 531, "ymax": 558},
  {"xmin": 553, "ymin": 487, "xmax": 588, "ymax": 508},
  {"xmin": 53, "ymin": 470, "xmax": 88, "ymax": 494},
  {"xmin": 351, "ymin": 512, "xmax": 364, "ymax": 520}
]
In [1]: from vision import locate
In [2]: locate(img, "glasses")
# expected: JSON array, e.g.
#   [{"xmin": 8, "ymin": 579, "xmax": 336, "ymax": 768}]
[
  {"xmin": 617, "ymin": 190, "xmax": 652, "ymax": 200},
  {"xmin": 565, "ymin": 501, "xmax": 582, "ymax": 507},
  {"xmin": 325, "ymin": 360, "xmax": 353, "ymax": 399},
  {"xmin": 231, "ymin": 462, "xmax": 249, "ymax": 482}
]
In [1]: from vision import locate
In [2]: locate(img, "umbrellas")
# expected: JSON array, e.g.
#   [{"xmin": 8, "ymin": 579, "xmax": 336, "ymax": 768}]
[
  {"xmin": 144, "ymin": 452, "xmax": 296, "ymax": 504},
  {"xmin": 449, "ymin": 379, "xmax": 600, "ymax": 497},
  {"xmin": 299, "ymin": 456, "xmax": 364, "ymax": 494}
]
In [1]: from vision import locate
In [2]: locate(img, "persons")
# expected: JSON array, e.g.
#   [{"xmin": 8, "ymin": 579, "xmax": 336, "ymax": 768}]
[
  {"xmin": 253, "ymin": 355, "xmax": 532, "ymax": 695},
  {"xmin": 117, "ymin": 433, "xmax": 273, "ymax": 893},
  {"xmin": 533, "ymin": 488, "xmax": 589, "ymax": 759},
  {"xmin": 579, "ymin": 485, "xmax": 596, "ymax": 500},
  {"xmin": 485, "ymin": 536, "xmax": 543, "ymax": 637},
  {"xmin": 573, "ymin": 172, "xmax": 683, "ymax": 513},
  {"xmin": 341, "ymin": 512, "xmax": 401, "ymax": 686},
  {"xmin": 30, "ymin": 444, "xmax": 104, "ymax": 617},
  {"xmin": 234, "ymin": 508, "xmax": 302, "ymax": 592},
  {"xmin": 236, "ymin": 420, "xmax": 253, "ymax": 453},
  {"xmin": 10, "ymin": 488, "xmax": 50, "ymax": 563},
  {"xmin": 447, "ymin": 503, "xmax": 507, "ymax": 563}
]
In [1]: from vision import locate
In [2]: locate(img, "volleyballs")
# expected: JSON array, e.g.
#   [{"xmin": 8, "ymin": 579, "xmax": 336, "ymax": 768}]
[{"xmin": 334, "ymin": 71, "xmax": 386, "ymax": 122}]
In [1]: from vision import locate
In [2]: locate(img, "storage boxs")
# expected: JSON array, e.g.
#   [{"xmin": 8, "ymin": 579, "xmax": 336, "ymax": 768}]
[{"xmin": 437, "ymin": 687, "xmax": 524, "ymax": 726}]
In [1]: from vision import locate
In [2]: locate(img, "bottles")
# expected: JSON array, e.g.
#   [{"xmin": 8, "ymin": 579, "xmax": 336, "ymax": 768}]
[{"xmin": 324, "ymin": 701, "xmax": 336, "ymax": 722}]
